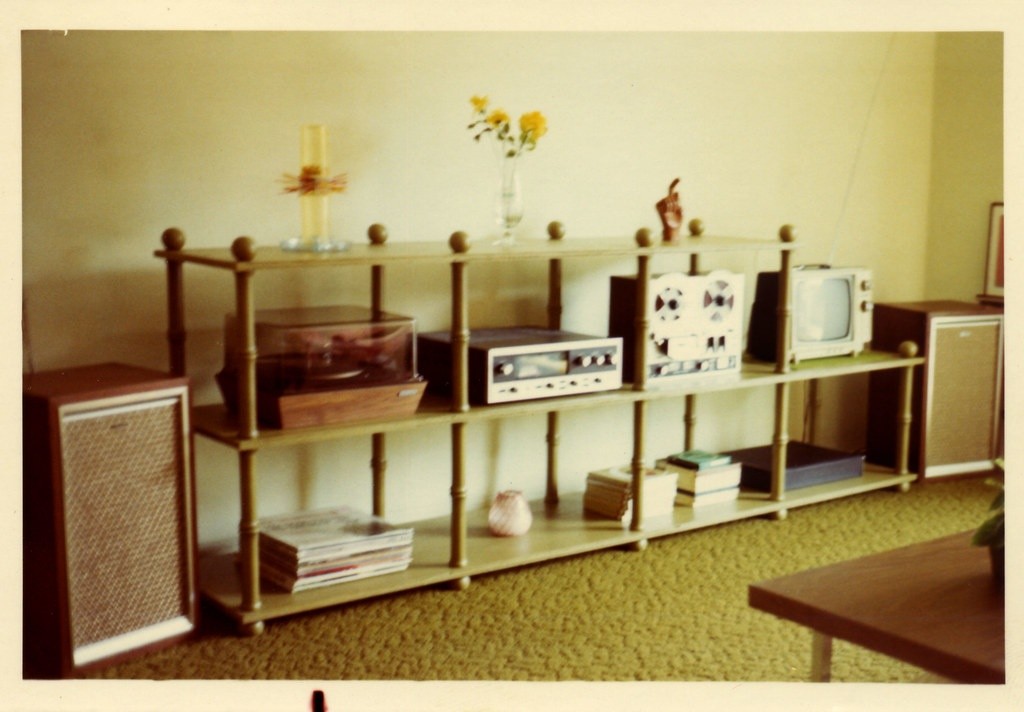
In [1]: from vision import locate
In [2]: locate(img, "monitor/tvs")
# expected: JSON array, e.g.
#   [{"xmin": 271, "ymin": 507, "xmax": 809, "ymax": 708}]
[{"xmin": 753, "ymin": 266, "xmax": 873, "ymax": 365}]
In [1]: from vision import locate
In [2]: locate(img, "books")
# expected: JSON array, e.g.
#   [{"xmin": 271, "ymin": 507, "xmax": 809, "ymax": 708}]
[
  {"xmin": 239, "ymin": 506, "xmax": 415, "ymax": 594},
  {"xmin": 582, "ymin": 451, "xmax": 742, "ymax": 522}
]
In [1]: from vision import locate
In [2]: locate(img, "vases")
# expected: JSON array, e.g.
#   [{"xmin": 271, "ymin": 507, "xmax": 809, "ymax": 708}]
[
  {"xmin": 498, "ymin": 159, "xmax": 525, "ymax": 242},
  {"xmin": 281, "ymin": 127, "xmax": 353, "ymax": 252}
]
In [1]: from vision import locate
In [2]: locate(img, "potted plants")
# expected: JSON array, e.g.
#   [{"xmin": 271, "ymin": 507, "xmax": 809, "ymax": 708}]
[{"xmin": 971, "ymin": 459, "xmax": 1004, "ymax": 587}]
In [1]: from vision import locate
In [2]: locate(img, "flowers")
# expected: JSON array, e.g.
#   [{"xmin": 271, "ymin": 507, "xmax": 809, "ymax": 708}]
[{"xmin": 468, "ymin": 96, "xmax": 548, "ymax": 215}]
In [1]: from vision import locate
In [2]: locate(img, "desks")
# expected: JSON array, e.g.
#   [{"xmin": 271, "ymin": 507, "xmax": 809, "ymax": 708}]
[{"xmin": 748, "ymin": 528, "xmax": 1005, "ymax": 686}]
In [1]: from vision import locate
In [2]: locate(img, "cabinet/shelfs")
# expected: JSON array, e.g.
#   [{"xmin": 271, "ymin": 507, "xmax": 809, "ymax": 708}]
[
  {"xmin": 23, "ymin": 362, "xmax": 198, "ymax": 679},
  {"xmin": 156, "ymin": 219, "xmax": 927, "ymax": 637},
  {"xmin": 872, "ymin": 300, "xmax": 1005, "ymax": 484}
]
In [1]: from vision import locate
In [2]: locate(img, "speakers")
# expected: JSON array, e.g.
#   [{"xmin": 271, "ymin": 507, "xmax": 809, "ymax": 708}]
[
  {"xmin": 19, "ymin": 362, "xmax": 199, "ymax": 678},
  {"xmin": 864, "ymin": 299, "xmax": 1004, "ymax": 481}
]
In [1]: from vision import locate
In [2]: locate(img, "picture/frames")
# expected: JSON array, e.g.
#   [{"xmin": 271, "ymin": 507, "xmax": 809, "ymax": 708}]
[{"xmin": 982, "ymin": 201, "xmax": 1005, "ymax": 305}]
